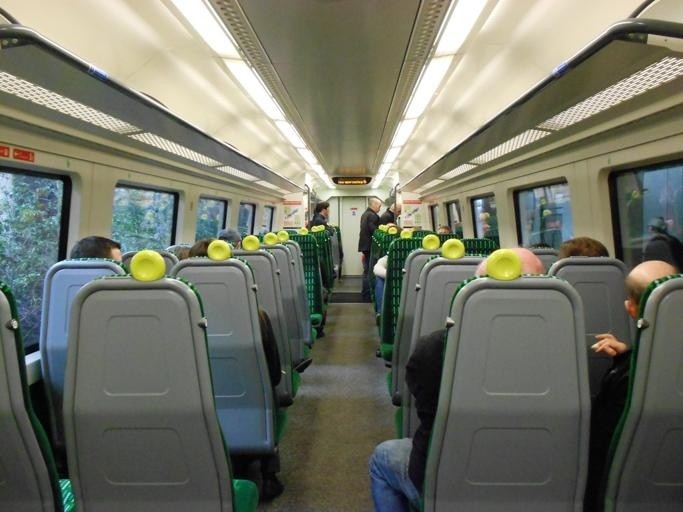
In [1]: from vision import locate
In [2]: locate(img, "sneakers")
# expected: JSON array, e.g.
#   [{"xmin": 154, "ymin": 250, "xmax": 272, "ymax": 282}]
[{"xmin": 262, "ymin": 475, "xmax": 282, "ymax": 496}]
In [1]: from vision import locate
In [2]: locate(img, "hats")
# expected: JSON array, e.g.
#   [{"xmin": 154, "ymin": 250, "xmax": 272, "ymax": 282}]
[
  {"xmin": 216, "ymin": 228, "xmax": 241, "ymax": 243},
  {"xmin": 649, "ymin": 216, "xmax": 667, "ymax": 231}
]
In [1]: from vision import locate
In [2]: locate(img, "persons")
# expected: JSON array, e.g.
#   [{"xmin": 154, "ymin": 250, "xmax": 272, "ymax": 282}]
[
  {"xmin": 559, "ymin": 236, "xmax": 610, "ymax": 258},
  {"xmin": 188, "ymin": 238, "xmax": 284, "ymax": 499},
  {"xmin": 174, "ymin": 246, "xmax": 190, "ymax": 261},
  {"xmin": 70, "ymin": 235, "xmax": 122, "ymax": 262},
  {"xmin": 368, "ymin": 247, "xmax": 544, "ymax": 512},
  {"xmin": 218, "ymin": 228, "xmax": 241, "ymax": 250},
  {"xmin": 381, "ymin": 203, "xmax": 401, "ymax": 225},
  {"xmin": 544, "ymin": 213, "xmax": 562, "ymax": 228},
  {"xmin": 386, "ymin": 225, "xmax": 403, "ymax": 234},
  {"xmin": 646, "ymin": 218, "xmax": 683, "ymax": 273},
  {"xmin": 373, "ymin": 254, "xmax": 388, "ymax": 315},
  {"xmin": 358, "ymin": 197, "xmax": 383, "ymax": 302},
  {"xmin": 312, "ymin": 202, "xmax": 338, "ymax": 279},
  {"xmin": 437, "ymin": 229, "xmax": 450, "ymax": 235},
  {"xmin": 585, "ymin": 258, "xmax": 678, "ymax": 512}
]
